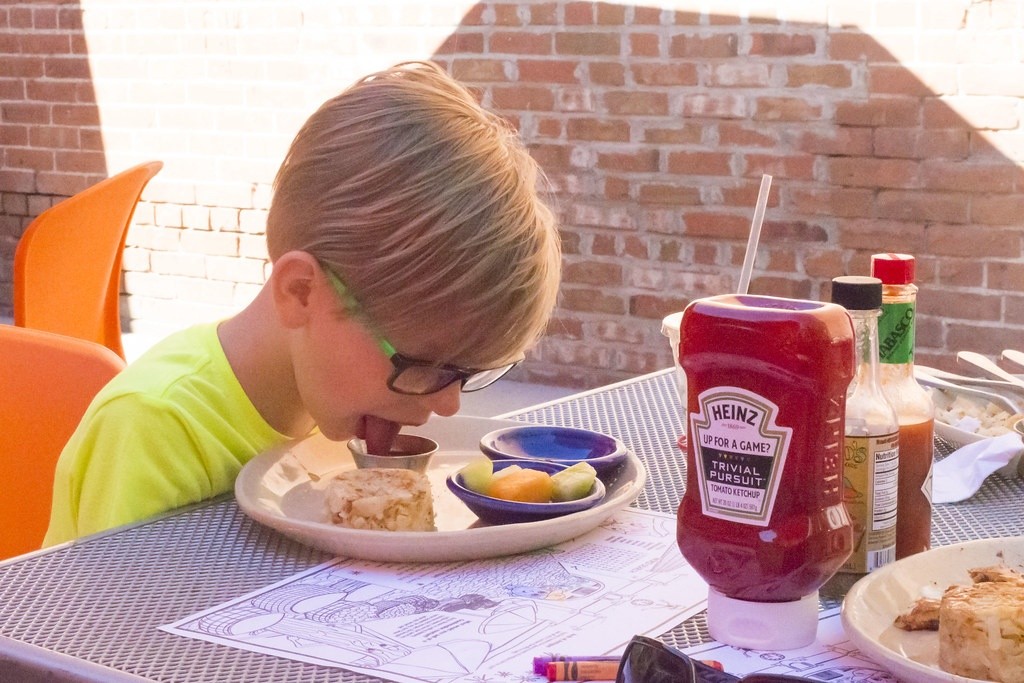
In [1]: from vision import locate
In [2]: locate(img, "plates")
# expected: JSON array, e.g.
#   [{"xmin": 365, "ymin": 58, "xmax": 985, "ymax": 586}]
[
  {"xmin": 931, "ymin": 374, "xmax": 1024, "ymax": 449},
  {"xmin": 236, "ymin": 414, "xmax": 647, "ymax": 561},
  {"xmin": 480, "ymin": 424, "xmax": 627, "ymax": 472},
  {"xmin": 841, "ymin": 537, "xmax": 1024, "ymax": 683},
  {"xmin": 446, "ymin": 459, "xmax": 607, "ymax": 526}
]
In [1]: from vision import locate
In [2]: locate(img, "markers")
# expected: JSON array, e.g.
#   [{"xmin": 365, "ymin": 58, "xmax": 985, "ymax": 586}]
[{"xmin": 532, "ymin": 656, "xmax": 724, "ymax": 681}]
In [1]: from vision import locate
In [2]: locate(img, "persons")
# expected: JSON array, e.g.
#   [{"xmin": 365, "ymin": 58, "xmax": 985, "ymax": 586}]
[{"xmin": 38, "ymin": 61, "xmax": 559, "ymax": 547}]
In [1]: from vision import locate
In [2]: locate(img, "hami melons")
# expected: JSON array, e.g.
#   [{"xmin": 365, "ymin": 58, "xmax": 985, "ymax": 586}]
[{"xmin": 487, "ymin": 468, "xmax": 551, "ymax": 505}]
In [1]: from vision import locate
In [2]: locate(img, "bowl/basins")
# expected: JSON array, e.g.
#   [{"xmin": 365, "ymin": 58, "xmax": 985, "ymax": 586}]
[
  {"xmin": 1014, "ymin": 419, "xmax": 1024, "ymax": 434},
  {"xmin": 348, "ymin": 433, "xmax": 440, "ymax": 474}
]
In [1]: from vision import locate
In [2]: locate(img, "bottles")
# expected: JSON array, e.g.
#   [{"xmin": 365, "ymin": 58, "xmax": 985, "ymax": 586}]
[
  {"xmin": 870, "ymin": 253, "xmax": 935, "ymax": 560},
  {"xmin": 675, "ymin": 293, "xmax": 856, "ymax": 651},
  {"xmin": 819, "ymin": 276, "xmax": 899, "ymax": 601}
]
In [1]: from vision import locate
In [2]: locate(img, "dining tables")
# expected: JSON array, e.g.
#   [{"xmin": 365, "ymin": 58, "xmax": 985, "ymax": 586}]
[{"xmin": 0, "ymin": 366, "xmax": 1024, "ymax": 682}]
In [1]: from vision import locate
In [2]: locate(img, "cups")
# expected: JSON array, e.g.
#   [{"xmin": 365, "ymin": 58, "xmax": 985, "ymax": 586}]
[{"xmin": 662, "ymin": 312, "xmax": 685, "ymax": 417}]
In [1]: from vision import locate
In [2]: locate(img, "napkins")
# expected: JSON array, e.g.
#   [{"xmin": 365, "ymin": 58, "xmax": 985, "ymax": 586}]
[{"xmin": 932, "ymin": 431, "xmax": 1024, "ymax": 504}]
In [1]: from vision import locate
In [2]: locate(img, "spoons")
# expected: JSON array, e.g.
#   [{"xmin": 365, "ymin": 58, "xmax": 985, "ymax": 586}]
[
  {"xmin": 914, "ymin": 375, "xmax": 1024, "ymax": 414},
  {"xmin": 914, "ymin": 364, "xmax": 1024, "ymax": 397}
]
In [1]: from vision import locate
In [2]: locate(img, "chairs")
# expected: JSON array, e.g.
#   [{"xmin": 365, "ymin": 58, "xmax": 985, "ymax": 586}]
[
  {"xmin": 0, "ymin": 324, "xmax": 128, "ymax": 562},
  {"xmin": 10, "ymin": 160, "xmax": 163, "ymax": 359}
]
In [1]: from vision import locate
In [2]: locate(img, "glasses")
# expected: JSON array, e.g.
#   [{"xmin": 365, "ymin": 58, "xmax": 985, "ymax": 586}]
[
  {"xmin": 611, "ymin": 632, "xmax": 827, "ymax": 682},
  {"xmin": 322, "ymin": 265, "xmax": 522, "ymax": 397}
]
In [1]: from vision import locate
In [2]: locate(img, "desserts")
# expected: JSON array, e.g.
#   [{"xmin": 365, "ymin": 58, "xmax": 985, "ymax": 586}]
[
  {"xmin": 934, "ymin": 580, "xmax": 1024, "ymax": 683},
  {"xmin": 326, "ymin": 468, "xmax": 437, "ymax": 532}
]
